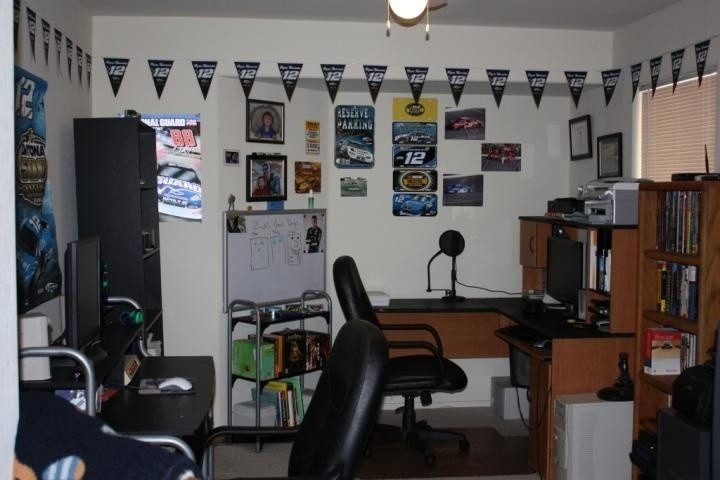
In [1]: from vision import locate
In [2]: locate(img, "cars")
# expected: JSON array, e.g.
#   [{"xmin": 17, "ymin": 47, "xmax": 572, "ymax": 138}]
[{"xmin": 296, "ymin": 162, "xmax": 320, "ymax": 192}]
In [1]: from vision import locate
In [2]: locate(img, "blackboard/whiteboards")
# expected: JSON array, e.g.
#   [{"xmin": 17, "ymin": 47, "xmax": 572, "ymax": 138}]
[{"xmin": 220, "ymin": 211, "xmax": 326, "ymax": 313}]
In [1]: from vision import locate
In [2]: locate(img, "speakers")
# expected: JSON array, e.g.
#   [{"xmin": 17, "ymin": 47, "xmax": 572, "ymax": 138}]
[{"xmin": 19, "ymin": 313, "xmax": 52, "ymax": 382}]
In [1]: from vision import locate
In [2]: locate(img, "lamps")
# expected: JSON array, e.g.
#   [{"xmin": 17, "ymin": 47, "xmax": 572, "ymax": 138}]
[{"xmin": 383, "ymin": 1, "xmax": 436, "ymax": 41}]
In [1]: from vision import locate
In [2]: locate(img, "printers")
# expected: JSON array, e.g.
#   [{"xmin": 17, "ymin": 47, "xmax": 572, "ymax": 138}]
[{"xmin": 565, "ymin": 174, "xmax": 659, "ymax": 226}]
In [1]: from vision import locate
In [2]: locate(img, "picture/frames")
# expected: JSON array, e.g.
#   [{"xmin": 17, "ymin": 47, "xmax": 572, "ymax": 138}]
[
  {"xmin": 594, "ymin": 130, "xmax": 626, "ymax": 181},
  {"xmin": 567, "ymin": 112, "xmax": 593, "ymax": 161},
  {"xmin": 245, "ymin": 96, "xmax": 288, "ymax": 146},
  {"xmin": 244, "ymin": 152, "xmax": 289, "ymax": 203}
]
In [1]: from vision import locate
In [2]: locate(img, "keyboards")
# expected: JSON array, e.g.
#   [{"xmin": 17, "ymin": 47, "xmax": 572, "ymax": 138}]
[{"xmin": 498, "ymin": 324, "xmax": 549, "ymax": 344}]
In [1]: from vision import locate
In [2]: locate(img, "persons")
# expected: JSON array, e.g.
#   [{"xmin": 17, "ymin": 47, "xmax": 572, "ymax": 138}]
[
  {"xmin": 264, "ymin": 163, "xmax": 280, "ymax": 196},
  {"xmin": 257, "ymin": 112, "xmax": 279, "ymax": 139},
  {"xmin": 305, "ymin": 215, "xmax": 321, "ymax": 252},
  {"xmin": 232, "ymin": 217, "xmax": 245, "ymax": 232},
  {"xmin": 253, "ymin": 176, "xmax": 270, "ymax": 197}
]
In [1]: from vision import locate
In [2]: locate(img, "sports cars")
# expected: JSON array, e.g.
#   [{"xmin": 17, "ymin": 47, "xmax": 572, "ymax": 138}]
[
  {"xmin": 156, "ymin": 164, "xmax": 201, "ymax": 211},
  {"xmin": 400, "ymin": 194, "xmax": 437, "ymax": 215},
  {"xmin": 447, "ymin": 183, "xmax": 472, "ymax": 195},
  {"xmin": 395, "ymin": 130, "xmax": 435, "ymax": 144},
  {"xmin": 449, "ymin": 117, "xmax": 484, "ymax": 131},
  {"xmin": 337, "ymin": 136, "xmax": 373, "ymax": 165},
  {"xmin": 16, "ymin": 214, "xmax": 53, "ymax": 305},
  {"xmin": 487, "ymin": 150, "xmax": 512, "ymax": 162}
]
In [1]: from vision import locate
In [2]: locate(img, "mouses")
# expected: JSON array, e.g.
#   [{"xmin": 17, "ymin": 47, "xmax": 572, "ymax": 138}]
[
  {"xmin": 532, "ymin": 338, "xmax": 550, "ymax": 349},
  {"xmin": 158, "ymin": 376, "xmax": 194, "ymax": 392}
]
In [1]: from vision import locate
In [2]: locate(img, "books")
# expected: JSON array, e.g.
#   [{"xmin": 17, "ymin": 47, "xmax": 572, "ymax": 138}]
[
  {"xmin": 661, "ymin": 190, "xmax": 700, "ymax": 372},
  {"xmin": 236, "ymin": 329, "xmax": 330, "ymax": 427}
]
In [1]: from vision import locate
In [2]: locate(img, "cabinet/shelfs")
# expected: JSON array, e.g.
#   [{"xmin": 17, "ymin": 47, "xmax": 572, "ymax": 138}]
[
  {"xmin": 72, "ymin": 113, "xmax": 169, "ymax": 358},
  {"xmin": 627, "ymin": 175, "xmax": 720, "ymax": 478},
  {"xmin": 225, "ymin": 287, "xmax": 334, "ymax": 454}
]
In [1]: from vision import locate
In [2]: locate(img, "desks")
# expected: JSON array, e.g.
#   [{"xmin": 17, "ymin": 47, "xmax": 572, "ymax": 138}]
[
  {"xmin": 46, "ymin": 315, "xmax": 217, "ymax": 462},
  {"xmin": 369, "ymin": 216, "xmax": 637, "ymax": 479}
]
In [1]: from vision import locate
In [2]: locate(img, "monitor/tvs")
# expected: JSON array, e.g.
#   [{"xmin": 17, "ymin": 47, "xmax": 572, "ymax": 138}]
[
  {"xmin": 546, "ymin": 236, "xmax": 587, "ymax": 325},
  {"xmin": 65, "ymin": 235, "xmax": 106, "ymax": 373}
]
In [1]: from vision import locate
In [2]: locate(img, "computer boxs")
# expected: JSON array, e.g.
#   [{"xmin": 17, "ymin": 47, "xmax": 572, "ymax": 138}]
[{"xmin": 553, "ymin": 393, "xmax": 634, "ymax": 480}]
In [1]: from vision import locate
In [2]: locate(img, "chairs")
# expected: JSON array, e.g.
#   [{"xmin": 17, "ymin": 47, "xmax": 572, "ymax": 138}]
[
  {"xmin": 328, "ymin": 248, "xmax": 474, "ymax": 476},
  {"xmin": 195, "ymin": 318, "xmax": 397, "ymax": 478}
]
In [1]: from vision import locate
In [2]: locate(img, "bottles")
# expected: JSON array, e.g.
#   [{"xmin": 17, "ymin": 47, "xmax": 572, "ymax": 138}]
[{"xmin": 307, "ymin": 189, "xmax": 314, "ymax": 210}]
